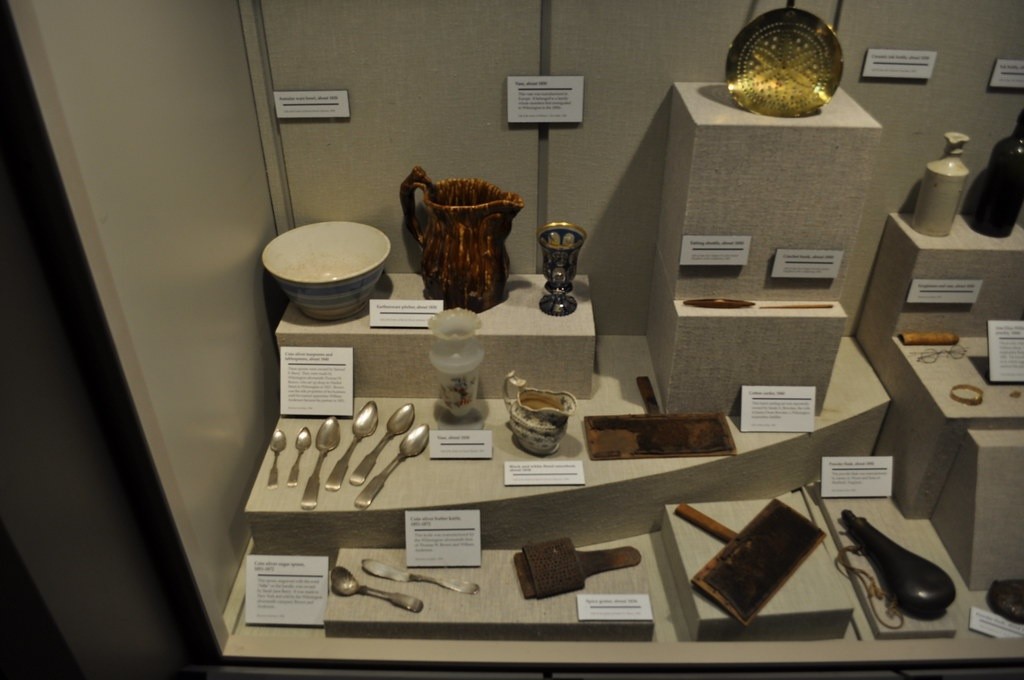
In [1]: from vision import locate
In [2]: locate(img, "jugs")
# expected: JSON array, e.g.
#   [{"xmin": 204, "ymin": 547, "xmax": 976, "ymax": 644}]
[
  {"xmin": 398, "ymin": 165, "xmax": 525, "ymax": 313},
  {"xmin": 502, "ymin": 369, "xmax": 578, "ymax": 456}
]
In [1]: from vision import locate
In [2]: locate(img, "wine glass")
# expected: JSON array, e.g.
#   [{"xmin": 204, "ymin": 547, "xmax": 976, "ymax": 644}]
[{"xmin": 537, "ymin": 220, "xmax": 588, "ymax": 316}]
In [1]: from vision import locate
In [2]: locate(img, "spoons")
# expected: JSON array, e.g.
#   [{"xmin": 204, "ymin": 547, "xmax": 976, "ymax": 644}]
[
  {"xmin": 354, "ymin": 423, "xmax": 430, "ymax": 509},
  {"xmin": 349, "ymin": 403, "xmax": 416, "ymax": 486},
  {"xmin": 331, "ymin": 566, "xmax": 424, "ymax": 613},
  {"xmin": 288, "ymin": 426, "xmax": 312, "ymax": 486},
  {"xmin": 267, "ymin": 429, "xmax": 287, "ymax": 490},
  {"xmin": 324, "ymin": 401, "xmax": 378, "ymax": 491},
  {"xmin": 301, "ymin": 417, "xmax": 341, "ymax": 510}
]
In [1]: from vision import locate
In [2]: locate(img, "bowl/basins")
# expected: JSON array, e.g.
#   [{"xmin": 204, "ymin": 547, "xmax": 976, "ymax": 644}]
[{"xmin": 262, "ymin": 220, "xmax": 391, "ymax": 321}]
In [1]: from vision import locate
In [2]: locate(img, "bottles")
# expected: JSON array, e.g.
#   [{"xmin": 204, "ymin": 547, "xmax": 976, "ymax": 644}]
[
  {"xmin": 971, "ymin": 108, "xmax": 1024, "ymax": 238},
  {"xmin": 426, "ymin": 307, "xmax": 486, "ymax": 433},
  {"xmin": 911, "ymin": 131, "xmax": 970, "ymax": 237}
]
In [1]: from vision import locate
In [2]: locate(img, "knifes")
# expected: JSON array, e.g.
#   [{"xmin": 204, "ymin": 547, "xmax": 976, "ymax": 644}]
[{"xmin": 362, "ymin": 559, "xmax": 480, "ymax": 594}]
